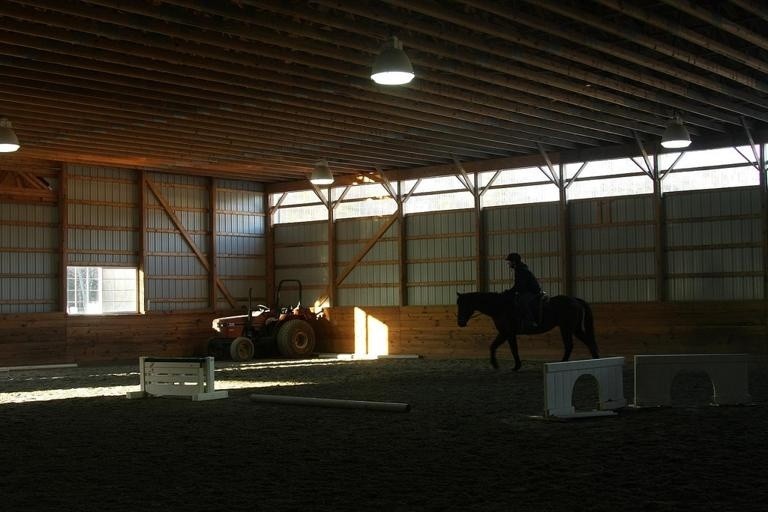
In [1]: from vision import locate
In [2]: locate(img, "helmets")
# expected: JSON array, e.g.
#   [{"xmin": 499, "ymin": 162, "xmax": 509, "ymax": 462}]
[{"xmin": 507, "ymin": 254, "xmax": 521, "ymax": 264}]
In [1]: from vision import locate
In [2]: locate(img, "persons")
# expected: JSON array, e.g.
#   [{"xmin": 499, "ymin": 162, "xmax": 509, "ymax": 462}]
[{"xmin": 504, "ymin": 253, "xmax": 542, "ymax": 329}]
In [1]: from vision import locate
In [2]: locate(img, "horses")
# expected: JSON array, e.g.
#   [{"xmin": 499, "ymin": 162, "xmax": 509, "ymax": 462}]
[{"xmin": 453, "ymin": 289, "xmax": 601, "ymax": 373}]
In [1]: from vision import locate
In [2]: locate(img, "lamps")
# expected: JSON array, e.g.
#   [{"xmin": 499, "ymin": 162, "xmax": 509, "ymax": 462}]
[
  {"xmin": 309, "ymin": 156, "xmax": 335, "ymax": 187},
  {"xmin": 660, "ymin": 109, "xmax": 692, "ymax": 150},
  {"xmin": 0, "ymin": 118, "xmax": 20, "ymax": 153},
  {"xmin": 369, "ymin": 25, "xmax": 416, "ymax": 87}
]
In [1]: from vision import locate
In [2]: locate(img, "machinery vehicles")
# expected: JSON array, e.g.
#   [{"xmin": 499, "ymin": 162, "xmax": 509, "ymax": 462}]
[{"xmin": 210, "ymin": 279, "xmax": 319, "ymax": 362}]
